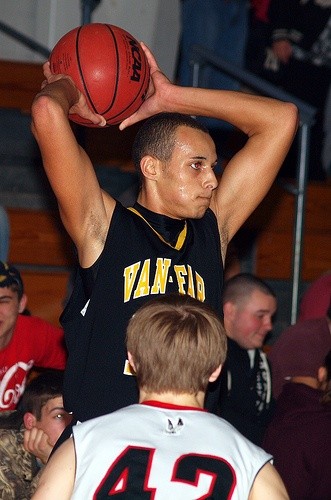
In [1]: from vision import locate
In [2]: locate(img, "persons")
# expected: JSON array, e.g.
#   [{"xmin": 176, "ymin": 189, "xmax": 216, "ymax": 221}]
[
  {"xmin": 0, "ymin": 261, "xmax": 65, "ymax": 411},
  {"xmin": 30, "ymin": 293, "xmax": 289, "ymax": 499},
  {"xmin": 261, "ymin": 304, "xmax": 331, "ymax": 500},
  {"xmin": 29, "ymin": 40, "xmax": 300, "ymax": 464},
  {"xmin": 177, "ymin": 1, "xmax": 331, "ymax": 192},
  {"xmin": 216, "ymin": 274, "xmax": 319, "ymax": 444},
  {"xmin": 0, "ymin": 372, "xmax": 72, "ymax": 500}
]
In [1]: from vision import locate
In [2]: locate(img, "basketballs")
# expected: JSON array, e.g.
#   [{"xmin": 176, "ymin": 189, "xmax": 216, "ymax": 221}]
[{"xmin": 48, "ymin": 24, "xmax": 149, "ymax": 128}]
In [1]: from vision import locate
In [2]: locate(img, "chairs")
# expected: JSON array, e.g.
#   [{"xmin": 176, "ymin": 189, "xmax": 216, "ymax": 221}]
[{"xmin": 1, "ymin": 59, "xmax": 331, "ymax": 326}]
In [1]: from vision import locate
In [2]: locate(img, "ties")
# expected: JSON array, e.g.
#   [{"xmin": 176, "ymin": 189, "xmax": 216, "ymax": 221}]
[{"xmin": 248, "ymin": 350, "xmax": 256, "ymax": 370}]
